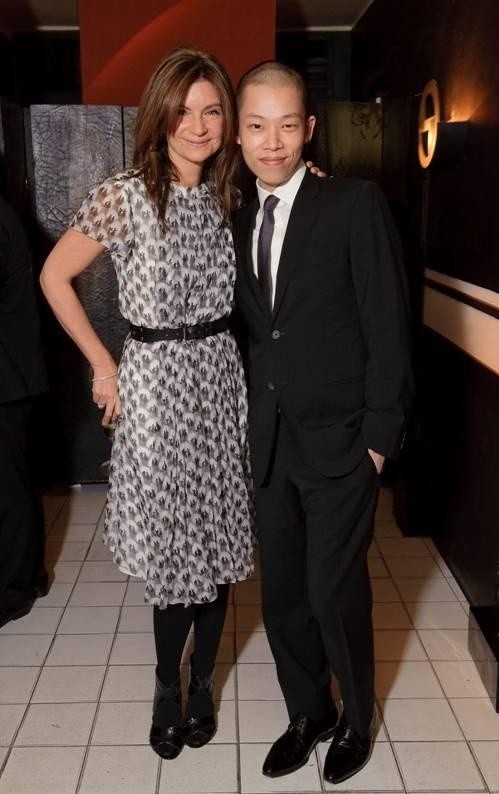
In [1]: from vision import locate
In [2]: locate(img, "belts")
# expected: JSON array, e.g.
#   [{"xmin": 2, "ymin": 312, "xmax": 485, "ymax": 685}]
[{"xmin": 130, "ymin": 318, "xmax": 228, "ymax": 342}]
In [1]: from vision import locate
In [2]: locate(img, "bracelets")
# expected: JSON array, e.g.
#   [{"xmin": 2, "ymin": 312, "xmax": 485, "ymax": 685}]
[{"xmin": 91, "ymin": 372, "xmax": 119, "ymax": 382}]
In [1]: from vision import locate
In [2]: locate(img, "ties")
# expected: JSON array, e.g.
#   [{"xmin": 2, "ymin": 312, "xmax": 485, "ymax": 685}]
[{"xmin": 257, "ymin": 195, "xmax": 280, "ymax": 313}]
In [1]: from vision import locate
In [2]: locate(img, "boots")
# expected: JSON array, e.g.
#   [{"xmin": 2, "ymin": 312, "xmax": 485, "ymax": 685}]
[
  {"xmin": 185, "ymin": 653, "xmax": 217, "ymax": 748},
  {"xmin": 149, "ymin": 664, "xmax": 184, "ymax": 760}
]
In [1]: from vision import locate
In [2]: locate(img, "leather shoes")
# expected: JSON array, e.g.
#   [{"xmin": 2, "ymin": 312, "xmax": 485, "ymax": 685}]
[
  {"xmin": 0, "ymin": 579, "xmax": 48, "ymax": 628},
  {"xmin": 262, "ymin": 701, "xmax": 339, "ymax": 777},
  {"xmin": 323, "ymin": 703, "xmax": 380, "ymax": 784}
]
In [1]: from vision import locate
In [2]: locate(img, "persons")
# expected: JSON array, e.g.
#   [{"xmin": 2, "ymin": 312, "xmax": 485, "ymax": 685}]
[
  {"xmin": 0, "ymin": 116, "xmax": 49, "ymax": 629},
  {"xmin": 232, "ymin": 59, "xmax": 414, "ymax": 783},
  {"xmin": 38, "ymin": 46, "xmax": 327, "ymax": 760}
]
